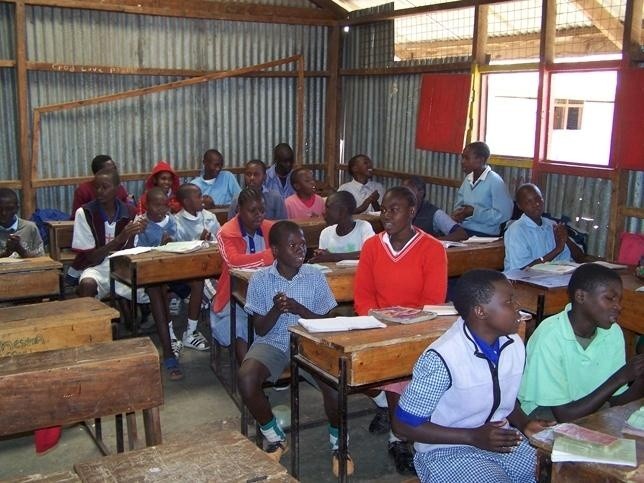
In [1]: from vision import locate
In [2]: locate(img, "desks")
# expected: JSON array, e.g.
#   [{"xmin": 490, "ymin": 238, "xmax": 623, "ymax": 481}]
[{"xmin": 0, "ymin": 221, "xmax": 644, "ymax": 483}]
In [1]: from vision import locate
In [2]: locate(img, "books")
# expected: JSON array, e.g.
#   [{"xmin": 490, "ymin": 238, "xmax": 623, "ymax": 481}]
[
  {"xmin": 624, "ymin": 404, "xmax": 644, "ymax": 437},
  {"xmin": 423, "ymin": 305, "xmax": 459, "ymax": 316},
  {"xmin": 441, "ymin": 241, "xmax": 468, "ymax": 249},
  {"xmin": 532, "ymin": 262, "xmax": 583, "ymax": 275},
  {"xmin": 549, "ymin": 433, "xmax": 638, "ymax": 469},
  {"xmin": 312, "ymin": 264, "xmax": 332, "ymax": 272},
  {"xmin": 297, "ymin": 315, "xmax": 387, "ymax": 334},
  {"xmin": 368, "ymin": 307, "xmax": 438, "ymax": 325},
  {"xmin": 552, "ymin": 418, "xmax": 620, "ymax": 454},
  {"xmin": 463, "ymin": 236, "xmax": 504, "ymax": 244}
]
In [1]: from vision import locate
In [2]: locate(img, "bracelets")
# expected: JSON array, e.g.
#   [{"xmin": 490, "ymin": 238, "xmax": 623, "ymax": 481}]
[{"xmin": 539, "ymin": 257, "xmax": 544, "ymax": 264}]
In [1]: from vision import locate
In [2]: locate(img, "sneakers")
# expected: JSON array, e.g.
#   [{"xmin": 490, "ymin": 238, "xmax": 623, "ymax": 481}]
[
  {"xmin": 265, "ymin": 439, "xmax": 289, "ymax": 462},
  {"xmin": 332, "ymin": 451, "xmax": 354, "ymax": 477},
  {"xmin": 163, "ymin": 331, "xmax": 212, "ymax": 379}
]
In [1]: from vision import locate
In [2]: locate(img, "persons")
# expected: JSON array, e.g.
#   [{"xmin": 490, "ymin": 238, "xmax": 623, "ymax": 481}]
[
  {"xmin": 190, "ymin": 143, "xmax": 468, "ymax": 264},
  {"xmin": 503, "ymin": 183, "xmax": 585, "ymax": 273},
  {"xmin": 515, "ymin": 260, "xmax": 643, "ymax": 424},
  {"xmin": 0, "ymin": 187, "xmax": 44, "ymax": 259},
  {"xmin": 235, "ymin": 216, "xmax": 353, "ymax": 477},
  {"xmin": 209, "ymin": 186, "xmax": 290, "ymax": 390},
  {"xmin": 66, "ymin": 154, "xmax": 223, "ymax": 379},
  {"xmin": 391, "ymin": 265, "xmax": 540, "ymax": 482},
  {"xmin": 352, "ymin": 186, "xmax": 448, "ymax": 475},
  {"xmin": 451, "ymin": 143, "xmax": 514, "ymax": 237}
]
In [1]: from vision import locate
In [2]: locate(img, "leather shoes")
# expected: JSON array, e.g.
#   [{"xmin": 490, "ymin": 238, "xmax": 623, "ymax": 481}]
[{"xmin": 369, "ymin": 406, "xmax": 417, "ymax": 476}]
